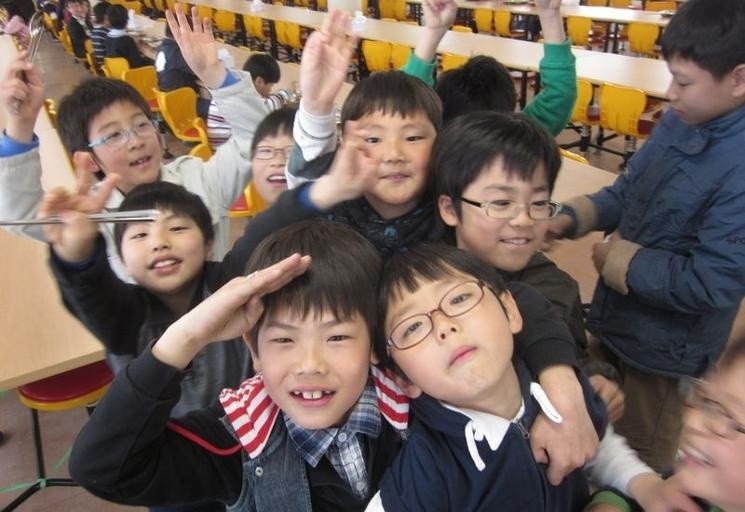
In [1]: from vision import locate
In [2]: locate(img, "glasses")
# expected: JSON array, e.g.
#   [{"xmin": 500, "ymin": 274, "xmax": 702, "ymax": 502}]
[
  {"xmin": 384, "ymin": 278, "xmax": 485, "ymax": 350},
  {"xmin": 87, "ymin": 118, "xmax": 165, "ymax": 150},
  {"xmin": 452, "ymin": 194, "xmax": 560, "ymax": 220},
  {"xmin": 250, "ymin": 144, "xmax": 294, "ymax": 160},
  {"xmin": 678, "ymin": 374, "xmax": 741, "ymax": 440}
]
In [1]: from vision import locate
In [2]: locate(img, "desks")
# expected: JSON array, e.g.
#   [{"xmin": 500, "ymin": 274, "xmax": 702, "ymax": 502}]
[{"xmin": 0, "ymin": 0, "xmax": 675, "ymax": 511}]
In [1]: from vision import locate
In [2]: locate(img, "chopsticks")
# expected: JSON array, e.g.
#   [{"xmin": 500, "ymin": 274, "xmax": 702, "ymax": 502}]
[{"xmin": 0, "ymin": 211, "xmax": 158, "ymax": 226}]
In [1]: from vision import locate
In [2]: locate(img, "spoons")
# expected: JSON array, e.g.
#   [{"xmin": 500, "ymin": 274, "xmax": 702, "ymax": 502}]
[{"xmin": 8, "ymin": 10, "xmax": 48, "ymax": 112}]
